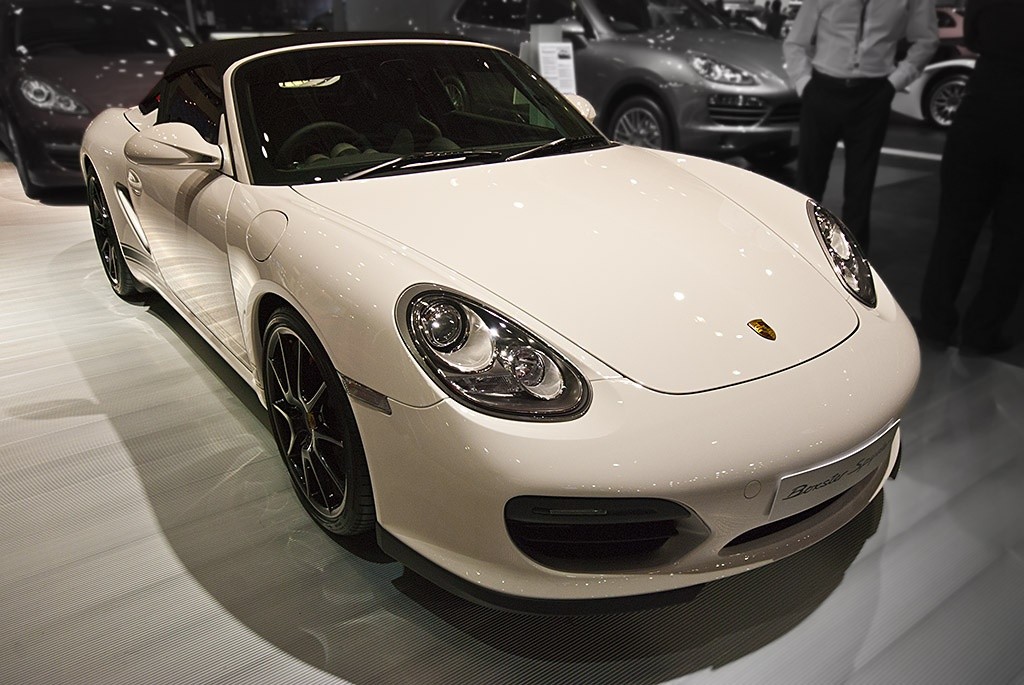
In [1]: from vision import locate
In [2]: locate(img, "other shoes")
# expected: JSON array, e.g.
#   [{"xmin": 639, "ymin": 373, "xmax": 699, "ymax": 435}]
[
  {"xmin": 959, "ymin": 333, "xmax": 1016, "ymax": 356},
  {"xmin": 918, "ymin": 313, "xmax": 948, "ymax": 352}
]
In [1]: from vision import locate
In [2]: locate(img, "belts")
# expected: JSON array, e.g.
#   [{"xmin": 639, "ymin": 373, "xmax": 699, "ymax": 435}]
[{"xmin": 813, "ymin": 68, "xmax": 885, "ymax": 88}]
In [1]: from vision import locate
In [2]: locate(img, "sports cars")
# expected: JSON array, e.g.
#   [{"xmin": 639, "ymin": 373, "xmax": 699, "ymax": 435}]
[
  {"xmin": 719, "ymin": 0, "xmax": 981, "ymax": 128},
  {"xmin": 80, "ymin": 30, "xmax": 924, "ymax": 618},
  {"xmin": 324, "ymin": 0, "xmax": 808, "ymax": 170},
  {"xmin": 0, "ymin": 0, "xmax": 203, "ymax": 199}
]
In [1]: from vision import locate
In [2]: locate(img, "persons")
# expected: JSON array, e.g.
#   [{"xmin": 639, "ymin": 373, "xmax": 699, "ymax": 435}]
[
  {"xmin": 909, "ymin": 0, "xmax": 1024, "ymax": 354},
  {"xmin": 782, "ymin": 0, "xmax": 939, "ymax": 254}
]
